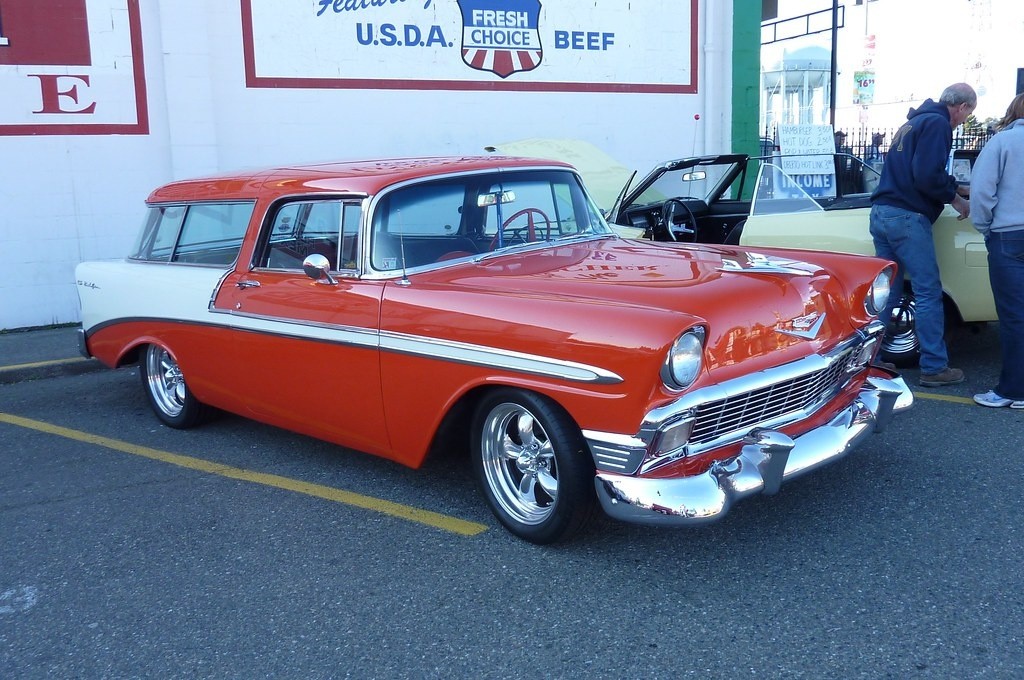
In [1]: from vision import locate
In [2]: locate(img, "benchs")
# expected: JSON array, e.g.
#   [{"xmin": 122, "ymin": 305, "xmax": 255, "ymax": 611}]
[{"xmin": 268, "ymin": 233, "xmax": 358, "ymax": 270}]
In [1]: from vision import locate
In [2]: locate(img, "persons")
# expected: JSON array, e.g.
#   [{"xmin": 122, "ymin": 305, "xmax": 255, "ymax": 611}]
[
  {"xmin": 835, "ymin": 131, "xmax": 847, "ymax": 154},
  {"xmin": 968, "ymin": 94, "xmax": 1024, "ymax": 410},
  {"xmin": 869, "ymin": 82, "xmax": 977, "ymax": 387},
  {"xmin": 865, "ymin": 133, "xmax": 882, "ymax": 161}
]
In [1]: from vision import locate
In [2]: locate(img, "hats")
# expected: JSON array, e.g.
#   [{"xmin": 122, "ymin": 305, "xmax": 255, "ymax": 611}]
[
  {"xmin": 835, "ymin": 131, "xmax": 847, "ymax": 136},
  {"xmin": 872, "ymin": 133, "xmax": 885, "ymax": 141}
]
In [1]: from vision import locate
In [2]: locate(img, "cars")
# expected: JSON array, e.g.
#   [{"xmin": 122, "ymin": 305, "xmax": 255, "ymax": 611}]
[
  {"xmin": 72, "ymin": 156, "xmax": 912, "ymax": 546},
  {"xmin": 484, "ymin": 134, "xmax": 1001, "ymax": 371}
]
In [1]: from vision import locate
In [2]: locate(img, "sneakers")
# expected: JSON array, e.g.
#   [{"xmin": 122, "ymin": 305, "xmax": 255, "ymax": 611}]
[
  {"xmin": 1010, "ymin": 401, "xmax": 1024, "ymax": 409},
  {"xmin": 920, "ymin": 368, "xmax": 965, "ymax": 387},
  {"xmin": 974, "ymin": 391, "xmax": 1011, "ymax": 407}
]
